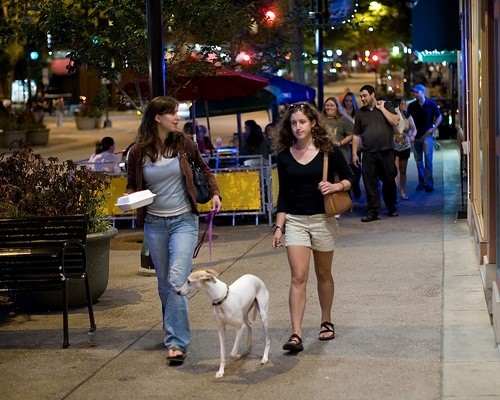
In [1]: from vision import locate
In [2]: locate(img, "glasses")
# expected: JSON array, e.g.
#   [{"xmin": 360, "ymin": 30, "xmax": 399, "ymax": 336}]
[{"xmin": 289, "ymin": 103, "xmax": 309, "ymax": 112}]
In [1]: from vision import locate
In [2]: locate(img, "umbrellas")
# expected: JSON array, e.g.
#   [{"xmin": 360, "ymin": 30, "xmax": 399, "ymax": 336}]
[
  {"xmin": 172, "ymin": 70, "xmax": 270, "ymax": 148},
  {"xmin": 258, "ymin": 70, "xmax": 316, "ymax": 121},
  {"xmin": 187, "ymin": 85, "xmax": 283, "ymax": 119}
]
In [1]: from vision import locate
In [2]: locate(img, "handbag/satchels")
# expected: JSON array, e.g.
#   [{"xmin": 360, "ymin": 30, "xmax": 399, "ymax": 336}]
[
  {"xmin": 180, "ymin": 134, "xmax": 211, "ymax": 204},
  {"xmin": 322, "ymin": 150, "xmax": 353, "ymax": 218},
  {"xmin": 380, "ymin": 100, "xmax": 406, "ymax": 135}
]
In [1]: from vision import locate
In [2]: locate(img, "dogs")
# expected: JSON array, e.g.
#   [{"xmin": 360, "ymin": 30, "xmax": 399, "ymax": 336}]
[{"xmin": 174, "ymin": 261, "xmax": 272, "ymax": 381}]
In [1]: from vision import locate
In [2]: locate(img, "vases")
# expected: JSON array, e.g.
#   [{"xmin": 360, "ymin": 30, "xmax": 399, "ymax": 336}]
[{"xmin": 55, "ymin": 109, "xmax": 62, "ymax": 128}]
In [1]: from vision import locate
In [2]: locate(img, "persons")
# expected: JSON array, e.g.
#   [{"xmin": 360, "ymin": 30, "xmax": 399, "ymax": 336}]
[
  {"xmin": 351, "ymin": 85, "xmax": 401, "ymax": 222},
  {"xmin": 238, "ymin": 119, "xmax": 275, "ymax": 164},
  {"xmin": 407, "ymin": 85, "xmax": 443, "ymax": 191},
  {"xmin": 53, "ymin": 96, "xmax": 64, "ymax": 128},
  {"xmin": 272, "ymin": 102, "xmax": 353, "ymax": 346},
  {"xmin": 122, "ymin": 97, "xmax": 223, "ymax": 361},
  {"xmin": 319, "ymin": 97, "xmax": 354, "ymax": 218},
  {"xmin": 392, "ymin": 98, "xmax": 417, "ymax": 197},
  {"xmin": 84, "ymin": 136, "xmax": 135, "ymax": 172},
  {"xmin": 341, "ymin": 93, "xmax": 364, "ymax": 198},
  {"xmin": 182, "ymin": 122, "xmax": 216, "ymax": 154}
]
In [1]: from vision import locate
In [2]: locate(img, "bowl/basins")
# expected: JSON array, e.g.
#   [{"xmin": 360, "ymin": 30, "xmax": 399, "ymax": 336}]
[{"xmin": 115, "ymin": 189, "xmax": 156, "ymax": 212}]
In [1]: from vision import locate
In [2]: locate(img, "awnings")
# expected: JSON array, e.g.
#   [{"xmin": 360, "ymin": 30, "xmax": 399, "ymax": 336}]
[{"xmin": 53, "ymin": 58, "xmax": 80, "ymax": 75}]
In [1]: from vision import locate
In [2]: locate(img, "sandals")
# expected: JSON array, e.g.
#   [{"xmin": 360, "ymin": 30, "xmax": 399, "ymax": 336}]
[
  {"xmin": 282, "ymin": 335, "xmax": 304, "ymax": 351},
  {"xmin": 319, "ymin": 321, "xmax": 334, "ymax": 340},
  {"xmin": 168, "ymin": 346, "xmax": 185, "ymax": 363}
]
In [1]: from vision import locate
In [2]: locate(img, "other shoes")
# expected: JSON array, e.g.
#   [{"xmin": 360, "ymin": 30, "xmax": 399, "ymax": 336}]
[
  {"xmin": 427, "ymin": 187, "xmax": 434, "ymax": 192},
  {"xmin": 390, "ymin": 210, "xmax": 398, "ymax": 216},
  {"xmin": 401, "ymin": 194, "xmax": 409, "ymax": 200},
  {"xmin": 416, "ymin": 184, "xmax": 423, "ymax": 190},
  {"xmin": 361, "ymin": 213, "xmax": 380, "ymax": 223}
]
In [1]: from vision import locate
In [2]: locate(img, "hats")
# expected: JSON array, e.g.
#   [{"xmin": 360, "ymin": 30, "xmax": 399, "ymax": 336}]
[{"xmin": 412, "ymin": 84, "xmax": 425, "ymax": 92}]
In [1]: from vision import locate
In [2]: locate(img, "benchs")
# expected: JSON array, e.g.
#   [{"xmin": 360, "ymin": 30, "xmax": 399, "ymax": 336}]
[
  {"xmin": 0, "ymin": 215, "xmax": 96, "ymax": 348},
  {"xmin": 75, "ymin": 147, "xmax": 279, "ymax": 228}
]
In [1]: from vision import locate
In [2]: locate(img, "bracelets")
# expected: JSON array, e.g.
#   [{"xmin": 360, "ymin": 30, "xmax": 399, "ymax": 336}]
[
  {"xmin": 274, "ymin": 226, "xmax": 285, "ymax": 234},
  {"xmin": 340, "ymin": 182, "xmax": 345, "ymax": 191}
]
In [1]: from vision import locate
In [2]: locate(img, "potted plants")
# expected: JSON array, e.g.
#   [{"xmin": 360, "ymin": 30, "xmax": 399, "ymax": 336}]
[
  {"xmin": 0, "ymin": 106, "xmax": 50, "ymax": 148},
  {"xmin": 0, "ymin": 139, "xmax": 118, "ymax": 309},
  {"xmin": 72, "ymin": 84, "xmax": 109, "ymax": 130}
]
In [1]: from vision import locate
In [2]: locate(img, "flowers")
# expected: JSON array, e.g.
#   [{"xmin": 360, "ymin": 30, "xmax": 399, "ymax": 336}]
[{"xmin": 52, "ymin": 95, "xmax": 65, "ymax": 113}]
